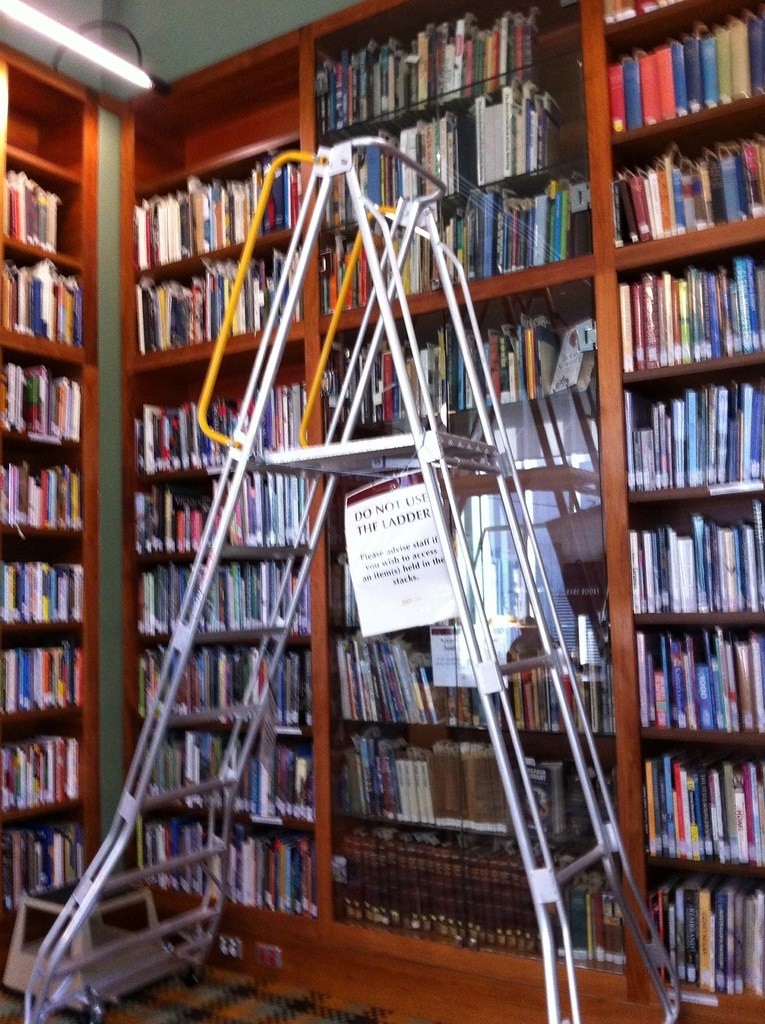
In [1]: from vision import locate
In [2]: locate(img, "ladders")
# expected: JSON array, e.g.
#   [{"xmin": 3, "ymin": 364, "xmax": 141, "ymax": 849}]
[{"xmin": 25, "ymin": 134, "xmax": 682, "ymax": 1024}]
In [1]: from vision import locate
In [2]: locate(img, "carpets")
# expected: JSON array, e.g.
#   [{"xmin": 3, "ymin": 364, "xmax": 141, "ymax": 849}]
[{"xmin": 0, "ymin": 969, "xmax": 434, "ymax": 1024}]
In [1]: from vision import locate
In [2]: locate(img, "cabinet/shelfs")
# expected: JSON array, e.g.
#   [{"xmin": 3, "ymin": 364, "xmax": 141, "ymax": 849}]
[
  {"xmin": 119, "ymin": 0, "xmax": 765, "ymax": 1024},
  {"xmin": 0, "ymin": 34, "xmax": 100, "ymax": 983}
]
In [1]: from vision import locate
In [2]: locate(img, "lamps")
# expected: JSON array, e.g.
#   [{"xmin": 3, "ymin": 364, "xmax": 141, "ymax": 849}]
[{"xmin": 0, "ymin": 0, "xmax": 172, "ymax": 100}]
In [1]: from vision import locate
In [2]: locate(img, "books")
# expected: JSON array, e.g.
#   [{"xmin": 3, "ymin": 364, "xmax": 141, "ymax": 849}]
[{"xmin": 0, "ymin": 0, "xmax": 765, "ymax": 996}]
